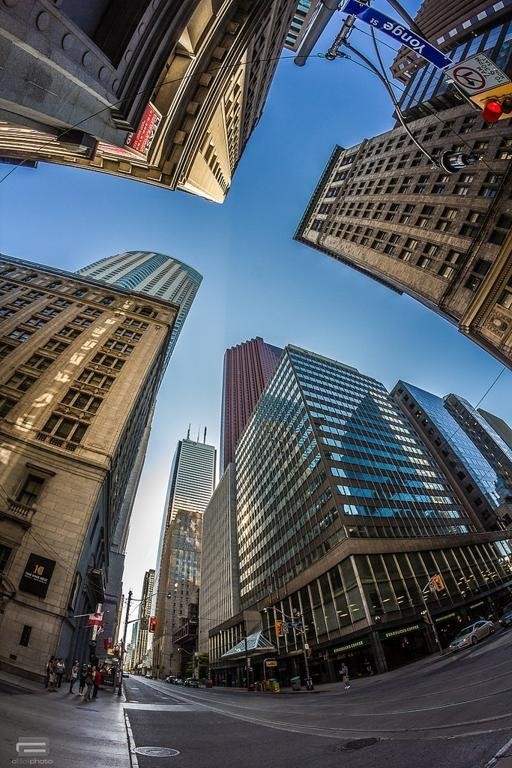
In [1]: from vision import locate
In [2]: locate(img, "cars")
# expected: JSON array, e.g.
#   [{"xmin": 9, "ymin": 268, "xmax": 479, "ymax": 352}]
[
  {"xmin": 164, "ymin": 676, "xmax": 199, "ymax": 687},
  {"xmin": 448, "ymin": 620, "xmax": 497, "ymax": 652},
  {"xmin": 499, "ymin": 602, "xmax": 512, "ymax": 630}
]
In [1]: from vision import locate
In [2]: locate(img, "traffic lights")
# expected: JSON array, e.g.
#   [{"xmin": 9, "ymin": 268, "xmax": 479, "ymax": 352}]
[
  {"xmin": 467, "ymin": 79, "xmax": 512, "ymax": 126},
  {"xmin": 148, "ymin": 615, "xmax": 159, "ymax": 633}
]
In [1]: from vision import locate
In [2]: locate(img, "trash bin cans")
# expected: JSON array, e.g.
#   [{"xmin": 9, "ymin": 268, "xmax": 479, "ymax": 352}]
[
  {"xmin": 269, "ymin": 678, "xmax": 277, "ymax": 691},
  {"xmin": 290, "ymin": 676, "xmax": 301, "ymax": 691}
]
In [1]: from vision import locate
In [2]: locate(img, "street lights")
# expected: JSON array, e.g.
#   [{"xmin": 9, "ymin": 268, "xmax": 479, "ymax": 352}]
[
  {"xmin": 262, "ymin": 605, "xmax": 314, "ymax": 692},
  {"xmin": 218, "ymin": 618, "xmax": 253, "ymax": 693},
  {"xmin": 177, "ymin": 647, "xmax": 195, "ymax": 680},
  {"xmin": 118, "ymin": 590, "xmax": 172, "ymax": 696},
  {"xmin": 433, "ymin": 575, "xmax": 446, "ymax": 592},
  {"xmin": 321, "ymin": 0, "xmax": 484, "ymax": 175}
]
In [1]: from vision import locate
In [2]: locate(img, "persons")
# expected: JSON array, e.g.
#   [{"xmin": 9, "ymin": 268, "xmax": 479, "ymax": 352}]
[
  {"xmin": 43, "ymin": 654, "xmax": 102, "ymax": 702},
  {"xmin": 339, "ymin": 662, "xmax": 351, "ymax": 689}
]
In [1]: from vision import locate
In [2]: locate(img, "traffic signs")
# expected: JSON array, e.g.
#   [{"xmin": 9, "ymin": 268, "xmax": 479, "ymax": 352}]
[{"xmin": 338, "ymin": 0, "xmax": 455, "ymax": 72}]
